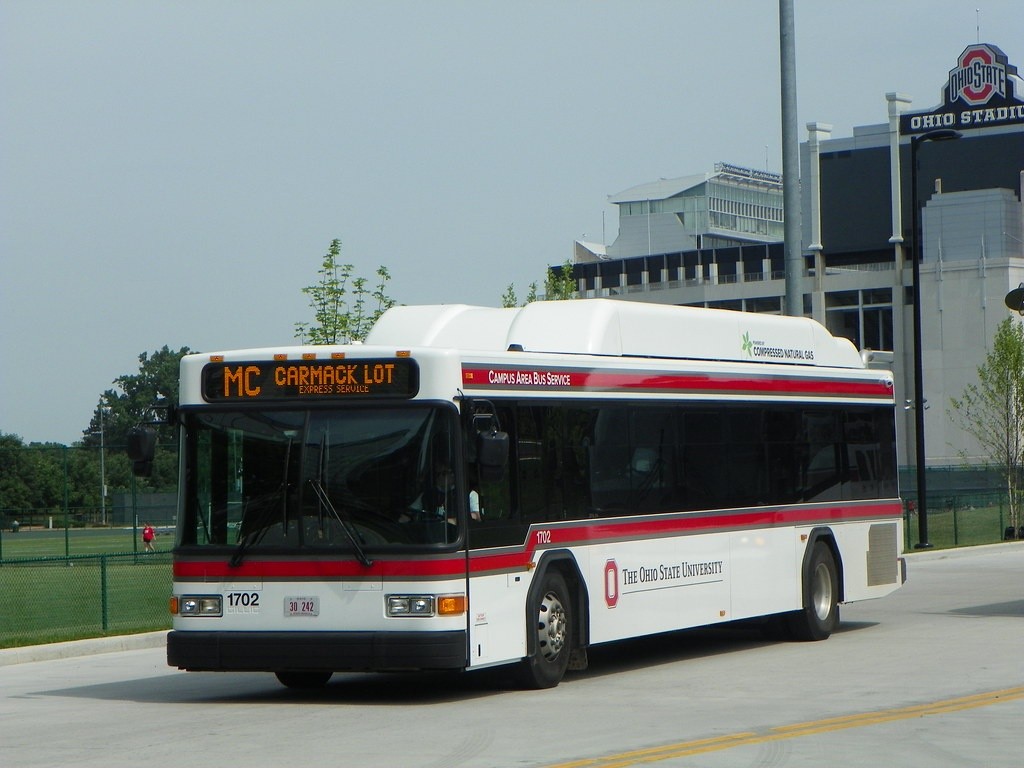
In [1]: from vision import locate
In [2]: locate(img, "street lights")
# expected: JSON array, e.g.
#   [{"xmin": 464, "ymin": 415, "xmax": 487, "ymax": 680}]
[
  {"xmin": 907, "ymin": 129, "xmax": 965, "ymax": 548},
  {"xmin": 98, "ymin": 403, "xmax": 112, "ymax": 526}
]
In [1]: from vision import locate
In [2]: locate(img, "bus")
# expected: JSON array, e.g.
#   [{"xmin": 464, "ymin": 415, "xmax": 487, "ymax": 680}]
[{"xmin": 125, "ymin": 296, "xmax": 912, "ymax": 693}]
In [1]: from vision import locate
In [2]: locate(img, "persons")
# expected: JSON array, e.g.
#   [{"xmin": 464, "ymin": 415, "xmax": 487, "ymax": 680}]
[
  {"xmin": 399, "ymin": 464, "xmax": 481, "ymax": 525},
  {"xmin": 140, "ymin": 521, "xmax": 157, "ymax": 555}
]
